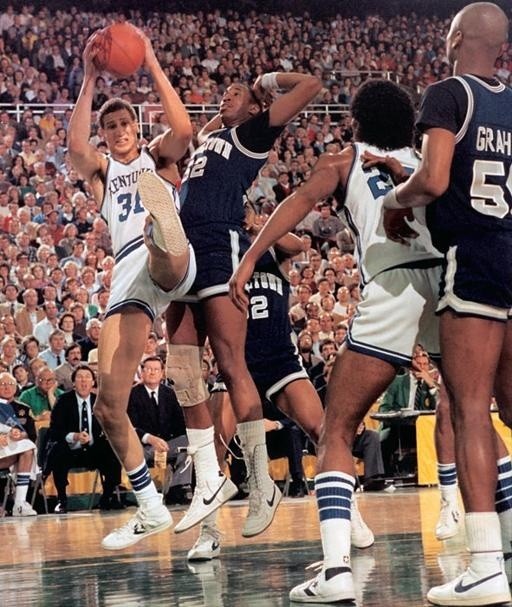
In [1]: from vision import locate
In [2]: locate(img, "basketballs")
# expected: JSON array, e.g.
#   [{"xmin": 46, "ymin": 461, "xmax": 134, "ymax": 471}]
[{"xmin": 90, "ymin": 24, "xmax": 145, "ymax": 77}]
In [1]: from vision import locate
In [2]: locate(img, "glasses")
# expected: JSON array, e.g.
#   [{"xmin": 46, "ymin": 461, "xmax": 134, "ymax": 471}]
[
  {"xmin": 0, "ymin": 381, "xmax": 15, "ymax": 385},
  {"xmin": 39, "ymin": 378, "xmax": 54, "ymax": 383},
  {"xmin": 143, "ymin": 368, "xmax": 160, "ymax": 373}
]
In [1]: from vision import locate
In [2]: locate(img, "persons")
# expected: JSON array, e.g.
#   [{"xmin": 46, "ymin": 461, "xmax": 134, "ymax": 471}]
[
  {"xmin": 66, "ymin": 18, "xmax": 199, "ymax": 551},
  {"xmin": 184, "ymin": 211, "xmax": 375, "ymax": 560},
  {"xmin": 0, "ymin": 0, "xmax": 510, "ymax": 518},
  {"xmin": 229, "ymin": 78, "xmax": 511, "ymax": 603},
  {"xmin": 434, "ymin": 383, "xmax": 461, "ymax": 541},
  {"xmin": 378, "ymin": 2, "xmax": 510, "ymax": 605},
  {"xmin": 163, "ymin": 70, "xmax": 322, "ymax": 537}
]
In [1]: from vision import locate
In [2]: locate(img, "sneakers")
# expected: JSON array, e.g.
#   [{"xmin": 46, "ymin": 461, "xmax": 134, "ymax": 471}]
[
  {"xmin": 100, "ymin": 506, "xmax": 173, "ymax": 551},
  {"xmin": 137, "ymin": 172, "xmax": 188, "ymax": 256},
  {"xmin": 288, "ymin": 567, "xmax": 355, "ymax": 603},
  {"xmin": 435, "ymin": 510, "xmax": 459, "ymax": 540},
  {"xmin": 174, "ymin": 473, "xmax": 240, "ymax": 533},
  {"xmin": 428, "ymin": 566, "xmax": 512, "ymax": 607},
  {"xmin": 12, "ymin": 501, "xmax": 38, "ymax": 516},
  {"xmin": 242, "ymin": 481, "xmax": 283, "ymax": 538},
  {"xmin": 186, "ymin": 524, "xmax": 222, "ymax": 560},
  {"xmin": 350, "ymin": 494, "xmax": 375, "ymax": 549}
]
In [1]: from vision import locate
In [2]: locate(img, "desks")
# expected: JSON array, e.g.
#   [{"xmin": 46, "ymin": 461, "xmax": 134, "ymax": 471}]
[{"xmin": 371, "ymin": 404, "xmax": 512, "ymax": 487}]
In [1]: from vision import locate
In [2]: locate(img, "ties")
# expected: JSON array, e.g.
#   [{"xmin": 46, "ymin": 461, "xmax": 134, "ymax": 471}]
[
  {"xmin": 151, "ymin": 391, "xmax": 157, "ymax": 406},
  {"xmin": 414, "ymin": 382, "xmax": 424, "ymax": 410},
  {"xmin": 81, "ymin": 403, "xmax": 90, "ymax": 453}
]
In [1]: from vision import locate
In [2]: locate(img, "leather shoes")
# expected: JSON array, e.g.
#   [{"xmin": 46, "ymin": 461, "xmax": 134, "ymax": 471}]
[
  {"xmin": 291, "ymin": 481, "xmax": 304, "ymax": 497},
  {"xmin": 363, "ymin": 478, "xmax": 385, "ymax": 490},
  {"xmin": 99, "ymin": 495, "xmax": 126, "ymax": 511},
  {"xmin": 55, "ymin": 503, "xmax": 67, "ymax": 513}
]
